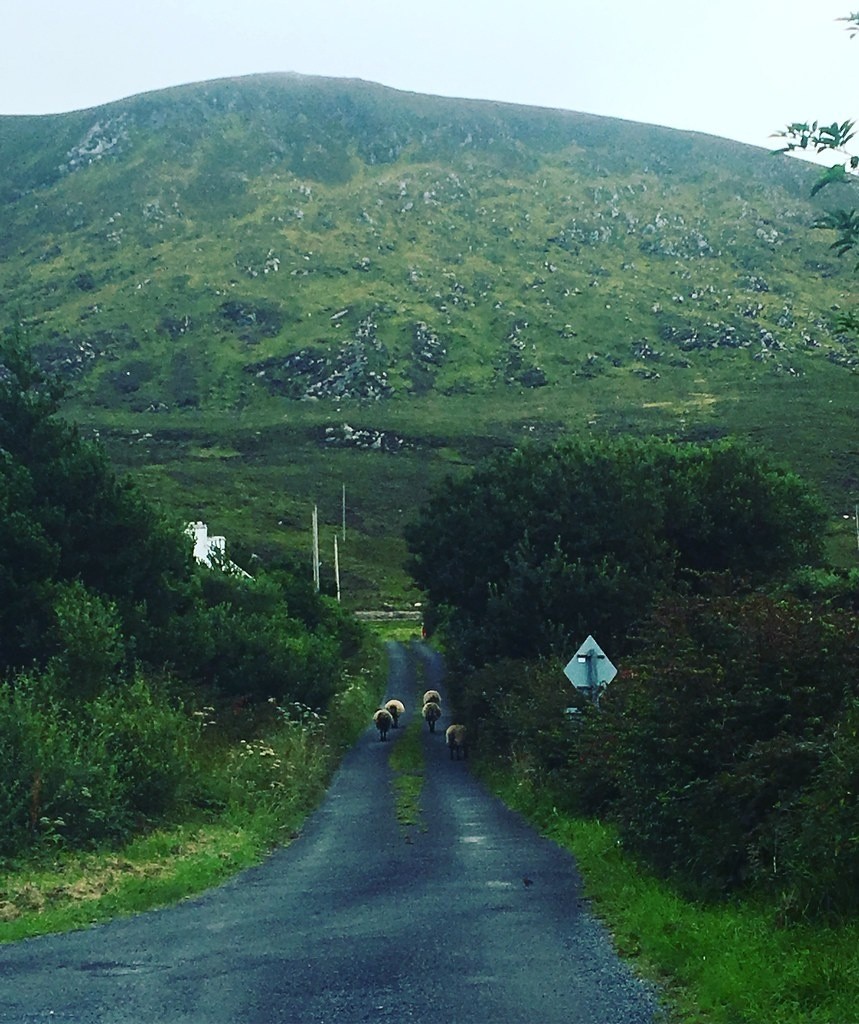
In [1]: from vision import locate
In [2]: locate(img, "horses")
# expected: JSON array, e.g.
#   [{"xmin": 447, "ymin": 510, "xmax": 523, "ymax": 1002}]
[{"xmin": 372, "ymin": 689, "xmax": 471, "ymax": 761}]
